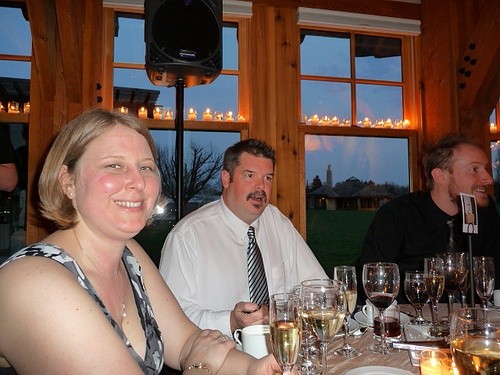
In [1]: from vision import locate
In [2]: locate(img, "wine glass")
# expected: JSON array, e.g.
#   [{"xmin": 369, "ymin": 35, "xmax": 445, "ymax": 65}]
[
  {"xmin": 289, "ymin": 279, "xmax": 321, "ymax": 375},
  {"xmin": 437, "ymin": 253, "xmax": 467, "ymax": 322},
  {"xmin": 301, "ymin": 280, "xmax": 347, "ymax": 375},
  {"xmin": 405, "ymin": 272, "xmax": 432, "ymax": 326},
  {"xmin": 363, "ymin": 263, "xmax": 402, "ymax": 355},
  {"xmin": 424, "ymin": 259, "xmax": 445, "ymax": 336},
  {"xmin": 271, "ymin": 293, "xmax": 302, "ymax": 375},
  {"xmin": 473, "ymin": 257, "xmax": 495, "ymax": 323},
  {"xmin": 334, "ymin": 266, "xmax": 360, "ymax": 357}
]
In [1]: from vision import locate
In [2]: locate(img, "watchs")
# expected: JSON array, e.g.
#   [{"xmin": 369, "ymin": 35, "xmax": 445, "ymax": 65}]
[{"xmin": 187, "ymin": 363, "xmax": 211, "ymax": 373}]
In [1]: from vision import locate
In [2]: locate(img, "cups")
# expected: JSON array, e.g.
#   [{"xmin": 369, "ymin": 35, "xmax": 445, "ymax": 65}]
[
  {"xmin": 450, "ymin": 309, "xmax": 500, "ymax": 375},
  {"xmin": 494, "ymin": 290, "xmax": 500, "ymax": 307},
  {"xmin": 362, "ymin": 299, "xmax": 397, "ymax": 321},
  {"xmin": 234, "ymin": 325, "xmax": 273, "ymax": 360},
  {"xmin": 373, "ymin": 305, "xmax": 401, "ymax": 342}
]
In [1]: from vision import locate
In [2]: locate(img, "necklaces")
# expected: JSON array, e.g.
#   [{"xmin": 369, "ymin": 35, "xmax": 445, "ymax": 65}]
[{"xmin": 72, "ymin": 229, "xmax": 127, "ymax": 318}]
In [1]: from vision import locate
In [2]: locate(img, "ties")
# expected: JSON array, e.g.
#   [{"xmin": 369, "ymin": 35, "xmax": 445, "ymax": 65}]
[
  {"xmin": 447, "ymin": 218, "xmax": 471, "ymax": 304},
  {"xmin": 247, "ymin": 226, "xmax": 270, "ymax": 309}
]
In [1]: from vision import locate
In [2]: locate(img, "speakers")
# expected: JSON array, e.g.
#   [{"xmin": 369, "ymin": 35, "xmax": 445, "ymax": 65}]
[{"xmin": 143, "ymin": 0, "xmax": 223, "ymax": 87}]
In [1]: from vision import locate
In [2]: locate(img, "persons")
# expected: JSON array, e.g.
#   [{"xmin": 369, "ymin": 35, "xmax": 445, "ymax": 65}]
[
  {"xmin": 348, "ymin": 131, "xmax": 500, "ymax": 306},
  {"xmin": 158, "ymin": 139, "xmax": 333, "ymax": 341},
  {"xmin": 0, "ymin": 110, "xmax": 302, "ymax": 375},
  {"xmin": 0, "ymin": 122, "xmax": 18, "ymax": 193}
]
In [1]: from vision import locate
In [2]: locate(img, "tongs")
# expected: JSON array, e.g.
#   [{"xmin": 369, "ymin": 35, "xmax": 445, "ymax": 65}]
[{"xmin": 393, "ymin": 339, "xmax": 450, "ymax": 353}]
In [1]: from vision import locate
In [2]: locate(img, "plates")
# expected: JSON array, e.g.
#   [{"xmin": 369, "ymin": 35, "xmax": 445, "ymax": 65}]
[
  {"xmin": 336, "ymin": 318, "xmax": 360, "ymax": 337},
  {"xmin": 354, "ymin": 312, "xmax": 411, "ymax": 328},
  {"xmin": 345, "ymin": 365, "xmax": 416, "ymax": 375}
]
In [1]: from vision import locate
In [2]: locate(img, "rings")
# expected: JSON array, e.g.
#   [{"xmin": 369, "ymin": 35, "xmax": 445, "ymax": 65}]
[{"xmin": 223, "ymin": 337, "xmax": 227, "ymax": 341}]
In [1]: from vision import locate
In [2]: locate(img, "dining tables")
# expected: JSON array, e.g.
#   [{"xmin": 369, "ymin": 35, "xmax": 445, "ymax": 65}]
[{"xmin": 296, "ymin": 303, "xmax": 500, "ymax": 375}]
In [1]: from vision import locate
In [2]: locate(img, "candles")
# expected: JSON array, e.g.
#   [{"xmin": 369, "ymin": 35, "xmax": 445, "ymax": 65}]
[
  {"xmin": 490, "ymin": 123, "xmax": 497, "ymax": 133},
  {"xmin": 310, "ymin": 114, "xmax": 411, "ymax": 128},
  {"xmin": 419, "ymin": 348, "xmax": 460, "ymax": 375},
  {"xmin": 117, "ymin": 106, "xmax": 246, "ymax": 122},
  {"xmin": 0, "ymin": 101, "xmax": 31, "ymax": 113}
]
